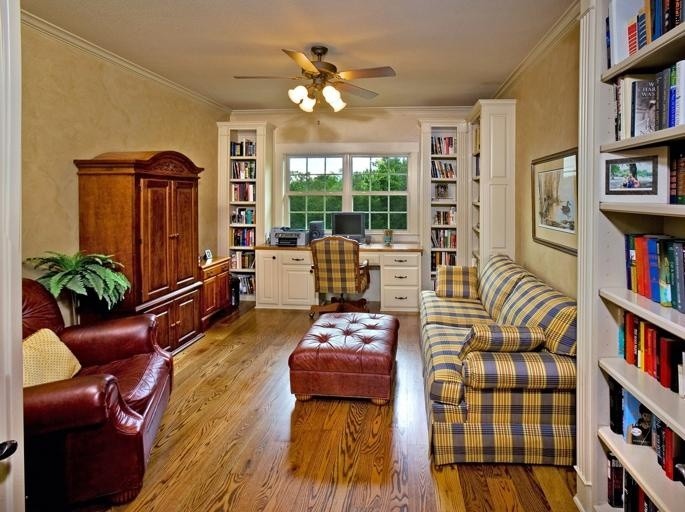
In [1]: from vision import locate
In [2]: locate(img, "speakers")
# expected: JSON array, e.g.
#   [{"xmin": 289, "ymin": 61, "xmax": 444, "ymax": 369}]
[{"xmin": 309, "ymin": 221, "xmax": 325, "ymax": 246}]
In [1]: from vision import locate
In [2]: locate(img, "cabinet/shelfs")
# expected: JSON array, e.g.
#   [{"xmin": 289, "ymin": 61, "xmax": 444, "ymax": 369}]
[
  {"xmin": 73, "ymin": 151, "xmax": 204, "ymax": 356},
  {"xmin": 254, "ymin": 247, "xmax": 423, "ymax": 313},
  {"xmin": 574, "ymin": 0, "xmax": 685, "ymax": 512},
  {"xmin": 216, "ymin": 120, "xmax": 277, "ymax": 301},
  {"xmin": 418, "ymin": 119, "xmax": 464, "ymax": 291},
  {"xmin": 200, "ymin": 257, "xmax": 232, "ymax": 333},
  {"xmin": 465, "ymin": 99, "xmax": 516, "ymax": 277}
]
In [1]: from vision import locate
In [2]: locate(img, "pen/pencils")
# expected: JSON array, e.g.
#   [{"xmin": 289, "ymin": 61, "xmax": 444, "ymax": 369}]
[{"xmin": 384, "ymin": 229, "xmax": 393, "ymax": 236}]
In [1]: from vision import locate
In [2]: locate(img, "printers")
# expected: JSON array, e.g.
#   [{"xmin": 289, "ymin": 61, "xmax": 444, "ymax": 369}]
[{"xmin": 271, "ymin": 226, "xmax": 309, "ymax": 247}]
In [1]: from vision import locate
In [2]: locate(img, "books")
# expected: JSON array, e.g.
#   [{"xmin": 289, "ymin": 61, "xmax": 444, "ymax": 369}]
[
  {"xmin": 609, "ymin": 377, "xmax": 621, "ymax": 435},
  {"xmin": 431, "ymin": 161, "xmax": 455, "ymax": 178},
  {"xmin": 230, "ymin": 138, "xmax": 256, "ymax": 157},
  {"xmin": 432, "ymin": 252, "xmax": 455, "ymax": 271},
  {"xmin": 622, "ymin": 387, "xmax": 652, "ymax": 446},
  {"xmin": 605, "ymin": 16, "xmax": 611, "ymax": 68},
  {"xmin": 434, "ymin": 212, "xmax": 451, "ymax": 224},
  {"xmin": 233, "ymin": 228, "xmax": 254, "ymax": 246},
  {"xmin": 430, "ymin": 231, "xmax": 456, "ymax": 247},
  {"xmin": 622, "ymin": 466, "xmax": 658, "ymax": 512},
  {"xmin": 231, "ymin": 207, "xmax": 255, "ymax": 224},
  {"xmin": 651, "ymin": 414, "xmax": 685, "ymax": 486},
  {"xmin": 232, "ymin": 161, "xmax": 256, "ymax": 179},
  {"xmin": 608, "ymin": 60, "xmax": 684, "ymax": 141},
  {"xmin": 617, "ymin": 308, "xmax": 685, "ymax": 399},
  {"xmin": 627, "ymin": 1, "xmax": 685, "ymax": 55},
  {"xmin": 238, "ymin": 275, "xmax": 254, "ymax": 294},
  {"xmin": 670, "ymin": 149, "xmax": 685, "ymax": 203},
  {"xmin": 625, "ymin": 234, "xmax": 685, "ymax": 314},
  {"xmin": 231, "ymin": 183, "xmax": 256, "ymax": 202},
  {"xmin": 607, "ymin": 451, "xmax": 625, "ymax": 508},
  {"xmin": 231, "ymin": 251, "xmax": 254, "ymax": 269},
  {"xmin": 431, "ymin": 136, "xmax": 454, "ymax": 155}
]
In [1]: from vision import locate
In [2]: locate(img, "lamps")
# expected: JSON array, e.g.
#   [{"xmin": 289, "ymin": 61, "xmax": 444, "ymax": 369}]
[{"xmin": 287, "ymin": 76, "xmax": 348, "ymax": 113}]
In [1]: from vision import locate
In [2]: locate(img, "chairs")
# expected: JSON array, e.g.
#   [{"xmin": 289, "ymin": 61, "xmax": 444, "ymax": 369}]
[{"xmin": 309, "ymin": 235, "xmax": 370, "ymax": 318}]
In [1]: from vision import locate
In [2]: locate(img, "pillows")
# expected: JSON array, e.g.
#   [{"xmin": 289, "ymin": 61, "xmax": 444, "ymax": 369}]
[
  {"xmin": 457, "ymin": 323, "xmax": 546, "ymax": 361},
  {"xmin": 22, "ymin": 328, "xmax": 82, "ymax": 387},
  {"xmin": 436, "ymin": 265, "xmax": 479, "ymax": 299}
]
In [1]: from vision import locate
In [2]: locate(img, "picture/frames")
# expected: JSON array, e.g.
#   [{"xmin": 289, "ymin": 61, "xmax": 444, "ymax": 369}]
[
  {"xmin": 205, "ymin": 250, "xmax": 212, "ymax": 258},
  {"xmin": 600, "ymin": 146, "xmax": 670, "ymax": 203},
  {"xmin": 531, "ymin": 147, "xmax": 577, "ymax": 257}
]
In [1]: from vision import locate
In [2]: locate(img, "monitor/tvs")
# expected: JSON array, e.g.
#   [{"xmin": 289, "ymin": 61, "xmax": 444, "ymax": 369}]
[{"xmin": 331, "ymin": 212, "xmax": 365, "ymax": 246}]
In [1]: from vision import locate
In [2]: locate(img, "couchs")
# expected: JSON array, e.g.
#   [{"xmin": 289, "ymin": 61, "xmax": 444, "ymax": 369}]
[
  {"xmin": 22, "ymin": 278, "xmax": 173, "ymax": 507},
  {"xmin": 419, "ymin": 254, "xmax": 576, "ymax": 465},
  {"xmin": 288, "ymin": 312, "xmax": 399, "ymax": 405}
]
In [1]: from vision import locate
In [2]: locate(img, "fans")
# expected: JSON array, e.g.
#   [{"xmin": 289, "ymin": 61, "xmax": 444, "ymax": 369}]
[{"xmin": 234, "ymin": 46, "xmax": 396, "ymax": 113}]
